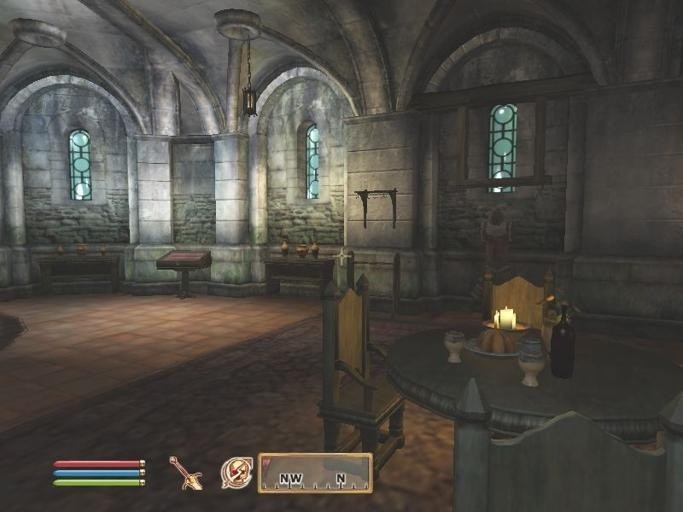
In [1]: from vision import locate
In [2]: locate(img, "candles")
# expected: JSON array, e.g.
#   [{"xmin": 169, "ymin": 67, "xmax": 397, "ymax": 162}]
[{"xmin": 499, "ymin": 305, "xmax": 513, "ymax": 328}]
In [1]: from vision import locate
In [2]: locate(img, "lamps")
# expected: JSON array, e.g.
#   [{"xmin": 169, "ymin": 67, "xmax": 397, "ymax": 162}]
[{"xmin": 240, "ymin": 33, "xmax": 258, "ymax": 120}]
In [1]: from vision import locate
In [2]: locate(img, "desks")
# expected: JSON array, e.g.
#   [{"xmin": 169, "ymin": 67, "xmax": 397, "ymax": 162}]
[
  {"xmin": 264, "ymin": 255, "xmax": 334, "ymax": 300},
  {"xmin": 382, "ymin": 322, "xmax": 681, "ymax": 445}
]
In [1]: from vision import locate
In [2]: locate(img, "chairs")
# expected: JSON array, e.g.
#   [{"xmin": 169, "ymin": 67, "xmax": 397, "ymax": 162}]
[
  {"xmin": 316, "ymin": 275, "xmax": 405, "ymax": 484},
  {"xmin": 482, "ymin": 264, "xmax": 554, "ymax": 329},
  {"xmin": 448, "ymin": 373, "xmax": 682, "ymax": 511}
]
